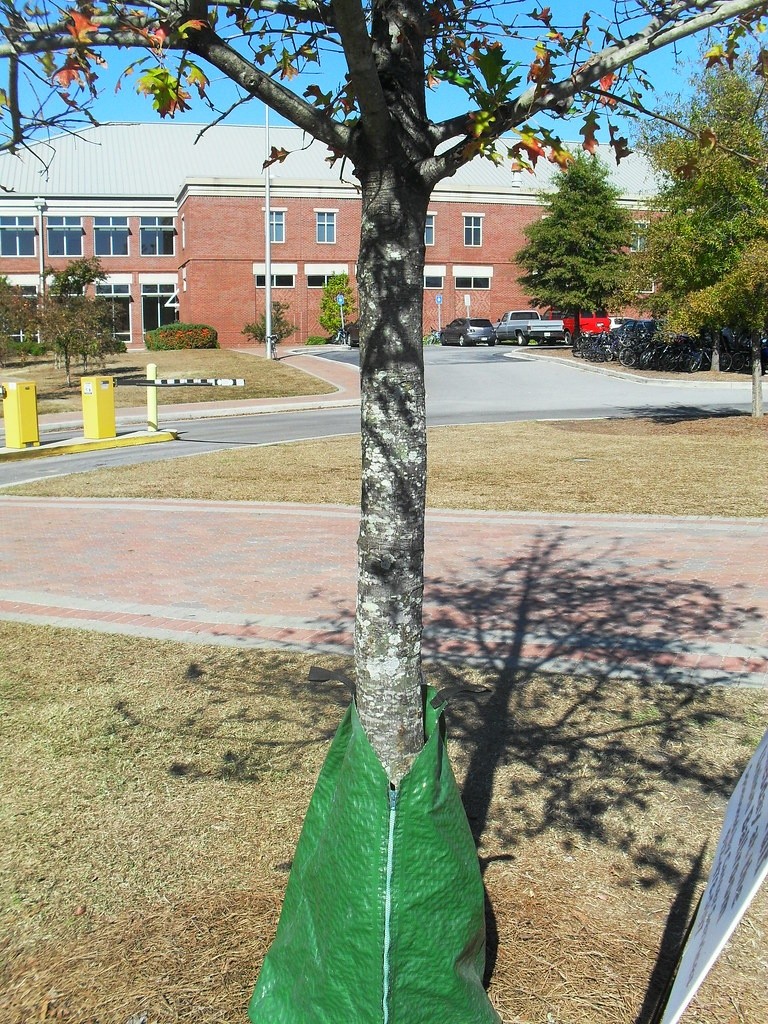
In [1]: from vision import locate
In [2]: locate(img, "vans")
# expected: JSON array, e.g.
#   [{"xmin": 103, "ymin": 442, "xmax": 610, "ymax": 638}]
[{"xmin": 541, "ymin": 305, "xmax": 611, "ymax": 346}]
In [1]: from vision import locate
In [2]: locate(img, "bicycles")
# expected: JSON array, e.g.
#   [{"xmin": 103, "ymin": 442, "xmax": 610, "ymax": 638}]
[
  {"xmin": 572, "ymin": 329, "xmax": 768, "ymax": 374},
  {"xmin": 332, "ymin": 328, "xmax": 347, "ymax": 347},
  {"xmin": 267, "ymin": 334, "xmax": 278, "ymax": 361}
]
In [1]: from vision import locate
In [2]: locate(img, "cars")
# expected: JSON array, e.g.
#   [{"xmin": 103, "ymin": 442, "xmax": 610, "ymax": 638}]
[
  {"xmin": 345, "ymin": 318, "xmax": 360, "ymax": 346},
  {"xmin": 608, "ymin": 316, "xmax": 656, "ymax": 334}
]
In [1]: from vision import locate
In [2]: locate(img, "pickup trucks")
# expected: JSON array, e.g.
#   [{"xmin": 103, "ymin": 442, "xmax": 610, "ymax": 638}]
[{"xmin": 493, "ymin": 310, "xmax": 565, "ymax": 346}]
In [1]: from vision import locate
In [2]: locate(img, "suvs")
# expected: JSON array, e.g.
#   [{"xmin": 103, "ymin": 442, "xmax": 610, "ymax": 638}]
[{"xmin": 440, "ymin": 317, "xmax": 496, "ymax": 347}]
